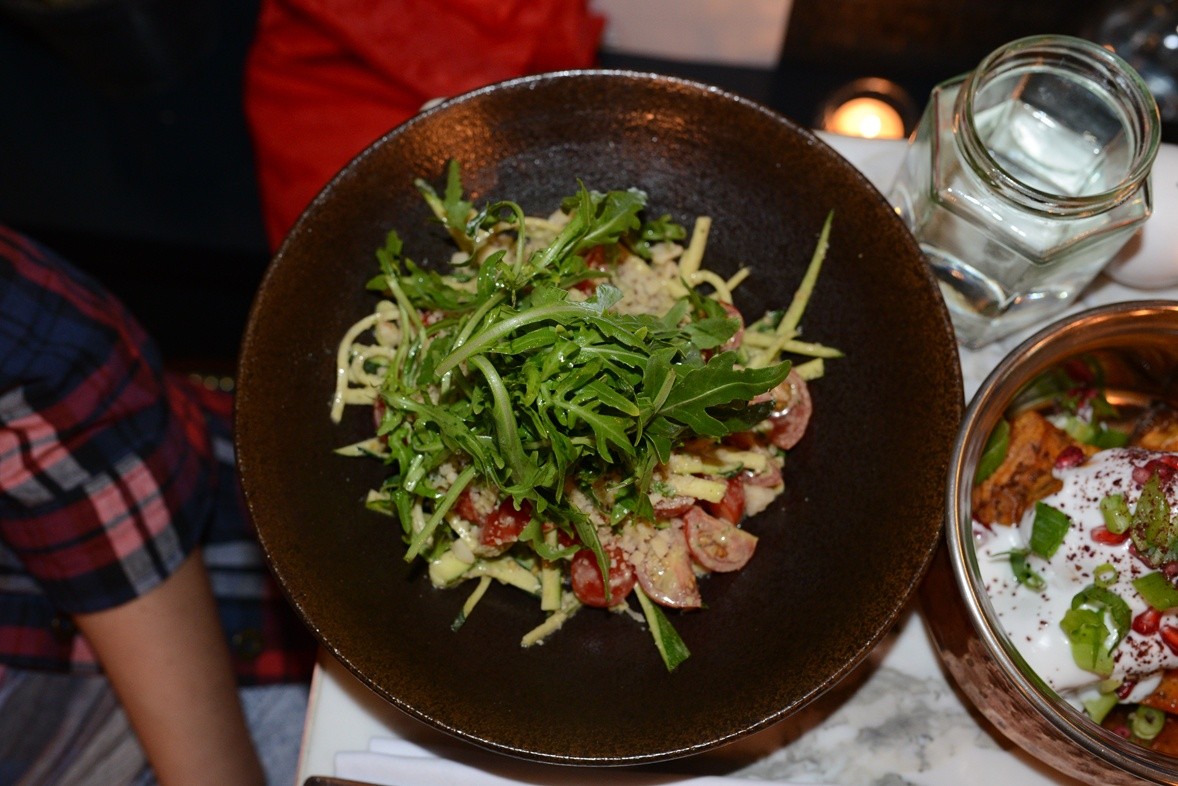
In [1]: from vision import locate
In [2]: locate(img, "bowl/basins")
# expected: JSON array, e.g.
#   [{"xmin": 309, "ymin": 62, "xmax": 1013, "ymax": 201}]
[{"xmin": 924, "ymin": 299, "xmax": 1177, "ymax": 783}]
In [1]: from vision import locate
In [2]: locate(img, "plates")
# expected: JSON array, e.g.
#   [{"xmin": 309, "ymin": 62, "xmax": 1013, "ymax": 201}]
[{"xmin": 231, "ymin": 70, "xmax": 966, "ymax": 771}]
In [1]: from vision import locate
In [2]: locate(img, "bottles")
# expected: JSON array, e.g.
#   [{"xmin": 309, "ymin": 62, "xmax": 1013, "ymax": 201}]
[{"xmin": 880, "ymin": 36, "xmax": 1165, "ymax": 347}]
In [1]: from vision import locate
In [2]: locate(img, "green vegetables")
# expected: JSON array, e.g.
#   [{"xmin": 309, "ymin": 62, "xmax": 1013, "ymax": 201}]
[{"xmin": 330, "ymin": 157, "xmax": 843, "ymax": 671}]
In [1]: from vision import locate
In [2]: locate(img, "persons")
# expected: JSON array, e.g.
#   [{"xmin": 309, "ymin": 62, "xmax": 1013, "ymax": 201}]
[
  {"xmin": 249, "ymin": 0, "xmax": 616, "ymax": 255},
  {"xmin": 6, "ymin": 220, "xmax": 318, "ymax": 786}
]
